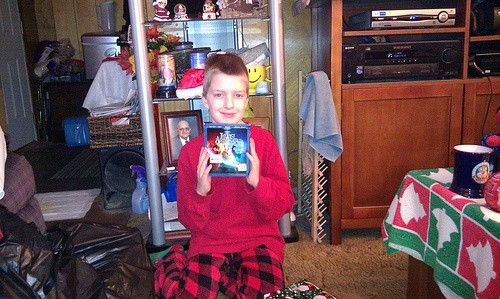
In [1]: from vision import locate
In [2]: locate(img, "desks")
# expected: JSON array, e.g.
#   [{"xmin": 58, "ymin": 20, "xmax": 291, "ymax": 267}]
[
  {"xmin": 37, "ymin": 79, "xmax": 93, "ymax": 144},
  {"xmin": 380, "ymin": 167, "xmax": 500, "ymax": 299}
]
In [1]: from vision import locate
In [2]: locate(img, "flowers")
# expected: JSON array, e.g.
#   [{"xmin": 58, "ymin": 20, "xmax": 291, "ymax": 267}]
[{"xmin": 118, "ymin": 27, "xmax": 181, "ymax": 85}]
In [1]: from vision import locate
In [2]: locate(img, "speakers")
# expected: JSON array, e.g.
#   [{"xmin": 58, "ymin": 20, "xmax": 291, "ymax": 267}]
[{"xmin": 97, "ymin": 146, "xmax": 147, "ymax": 210}]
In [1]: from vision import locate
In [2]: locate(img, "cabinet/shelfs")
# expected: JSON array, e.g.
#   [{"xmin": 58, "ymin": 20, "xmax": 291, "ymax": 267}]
[
  {"xmin": 129, "ymin": 0, "xmax": 299, "ymax": 253},
  {"xmin": 311, "ymin": 0, "xmax": 500, "ymax": 246}
]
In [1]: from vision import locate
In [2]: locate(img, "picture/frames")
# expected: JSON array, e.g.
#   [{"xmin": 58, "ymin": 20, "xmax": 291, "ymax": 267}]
[{"xmin": 160, "ymin": 109, "xmax": 204, "ymax": 167}]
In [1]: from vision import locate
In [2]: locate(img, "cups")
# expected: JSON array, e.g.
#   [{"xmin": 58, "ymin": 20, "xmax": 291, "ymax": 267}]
[{"xmin": 448, "ymin": 144, "xmax": 500, "ymax": 198}]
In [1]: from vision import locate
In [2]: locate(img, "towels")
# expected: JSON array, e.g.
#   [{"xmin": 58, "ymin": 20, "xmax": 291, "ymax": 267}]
[{"xmin": 297, "ymin": 70, "xmax": 344, "ymax": 163}]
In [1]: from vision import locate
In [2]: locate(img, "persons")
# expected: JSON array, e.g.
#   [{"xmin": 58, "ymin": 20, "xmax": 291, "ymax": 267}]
[
  {"xmin": 151, "ymin": 51, "xmax": 297, "ymax": 299},
  {"xmin": 212, "ymin": 144, "xmax": 242, "ymax": 166},
  {"xmin": 170, "ymin": 119, "xmax": 199, "ymax": 161}
]
